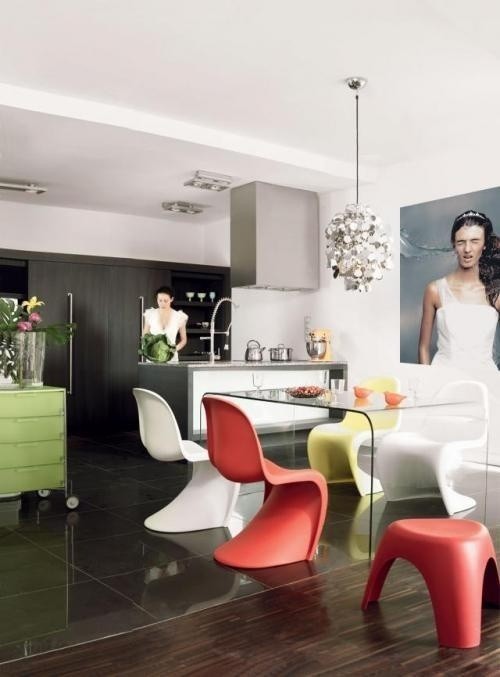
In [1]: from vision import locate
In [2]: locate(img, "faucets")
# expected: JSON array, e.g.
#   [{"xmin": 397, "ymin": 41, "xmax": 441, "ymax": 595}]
[{"xmin": 211, "ymin": 297, "xmax": 239, "ymax": 362}]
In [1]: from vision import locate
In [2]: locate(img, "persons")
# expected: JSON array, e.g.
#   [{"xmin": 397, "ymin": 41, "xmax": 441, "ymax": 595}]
[
  {"xmin": 419, "ymin": 210, "xmax": 500, "ymax": 371},
  {"xmin": 138, "ymin": 287, "xmax": 187, "ymax": 363}
]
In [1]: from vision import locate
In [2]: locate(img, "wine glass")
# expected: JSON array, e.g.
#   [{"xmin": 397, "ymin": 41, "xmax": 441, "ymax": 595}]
[
  {"xmin": 197, "ymin": 292, "xmax": 207, "ymax": 302},
  {"xmin": 209, "ymin": 291, "xmax": 216, "ymax": 302},
  {"xmin": 186, "ymin": 291, "xmax": 195, "ymax": 302}
]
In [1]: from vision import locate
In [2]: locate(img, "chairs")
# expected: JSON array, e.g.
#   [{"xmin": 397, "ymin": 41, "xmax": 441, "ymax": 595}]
[
  {"xmin": 305, "ymin": 375, "xmax": 398, "ymax": 497},
  {"xmin": 201, "ymin": 397, "xmax": 329, "ymax": 569},
  {"xmin": 375, "ymin": 381, "xmax": 490, "ymax": 515},
  {"xmin": 132, "ymin": 388, "xmax": 241, "ymax": 534}
]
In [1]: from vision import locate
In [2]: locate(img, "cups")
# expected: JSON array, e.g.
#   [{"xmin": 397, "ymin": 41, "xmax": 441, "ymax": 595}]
[
  {"xmin": 202, "ymin": 321, "xmax": 210, "ymax": 328},
  {"xmin": 322, "ymin": 390, "xmax": 332, "ymax": 404}
]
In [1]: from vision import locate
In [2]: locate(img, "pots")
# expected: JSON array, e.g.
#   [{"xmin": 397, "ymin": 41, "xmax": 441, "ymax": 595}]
[
  {"xmin": 305, "ymin": 341, "xmax": 328, "ymax": 361},
  {"xmin": 268, "ymin": 343, "xmax": 293, "ymax": 360}
]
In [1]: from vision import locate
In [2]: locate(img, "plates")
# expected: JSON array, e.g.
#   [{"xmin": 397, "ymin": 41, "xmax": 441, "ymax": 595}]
[{"xmin": 285, "ymin": 390, "xmax": 319, "ymax": 398}]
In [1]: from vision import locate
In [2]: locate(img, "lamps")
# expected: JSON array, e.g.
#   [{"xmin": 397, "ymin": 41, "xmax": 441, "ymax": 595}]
[
  {"xmin": 182, "ymin": 169, "xmax": 231, "ymax": 192},
  {"xmin": 324, "ymin": 77, "xmax": 393, "ymax": 292},
  {"xmin": 0, "ymin": 180, "xmax": 46, "ymax": 196},
  {"xmin": 161, "ymin": 200, "xmax": 203, "ymax": 216}
]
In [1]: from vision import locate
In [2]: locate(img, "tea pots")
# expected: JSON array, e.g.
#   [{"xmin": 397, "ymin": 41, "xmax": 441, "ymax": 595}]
[{"xmin": 244, "ymin": 339, "xmax": 266, "ymax": 361}]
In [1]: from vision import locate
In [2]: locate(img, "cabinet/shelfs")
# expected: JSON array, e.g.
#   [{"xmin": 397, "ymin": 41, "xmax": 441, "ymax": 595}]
[
  {"xmin": 28, "ymin": 259, "xmax": 171, "ymax": 438},
  {"xmin": 228, "ymin": 182, "xmax": 320, "ymax": 293},
  {"xmin": 171, "ymin": 268, "xmax": 232, "ymax": 363},
  {"xmin": 0, "ymin": 387, "xmax": 82, "ymax": 512},
  {"xmin": 0, "ymin": 257, "xmax": 29, "ymax": 325}
]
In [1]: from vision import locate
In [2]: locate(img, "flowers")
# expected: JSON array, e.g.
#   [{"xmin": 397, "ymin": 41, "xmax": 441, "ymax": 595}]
[{"xmin": 0, "ymin": 293, "xmax": 77, "ymax": 385}]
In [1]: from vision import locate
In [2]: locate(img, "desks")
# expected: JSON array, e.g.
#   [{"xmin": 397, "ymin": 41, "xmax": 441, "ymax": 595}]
[{"xmin": 203, "ymin": 384, "xmax": 490, "ymax": 562}]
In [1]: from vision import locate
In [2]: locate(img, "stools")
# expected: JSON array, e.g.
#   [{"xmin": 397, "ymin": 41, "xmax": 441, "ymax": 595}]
[{"xmin": 361, "ymin": 517, "xmax": 499, "ymax": 650}]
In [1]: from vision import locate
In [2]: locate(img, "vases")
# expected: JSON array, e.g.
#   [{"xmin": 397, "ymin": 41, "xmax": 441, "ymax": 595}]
[{"xmin": 11, "ymin": 331, "xmax": 46, "ymax": 388}]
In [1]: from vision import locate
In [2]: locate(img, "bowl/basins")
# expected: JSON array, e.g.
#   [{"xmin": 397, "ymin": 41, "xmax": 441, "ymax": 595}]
[
  {"xmin": 353, "ymin": 385, "xmax": 375, "ymax": 398},
  {"xmin": 382, "ymin": 390, "xmax": 406, "ymax": 404}
]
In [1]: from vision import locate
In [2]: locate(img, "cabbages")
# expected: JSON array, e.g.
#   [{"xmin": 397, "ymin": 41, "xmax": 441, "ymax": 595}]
[{"xmin": 139, "ymin": 334, "xmax": 175, "ymax": 363}]
